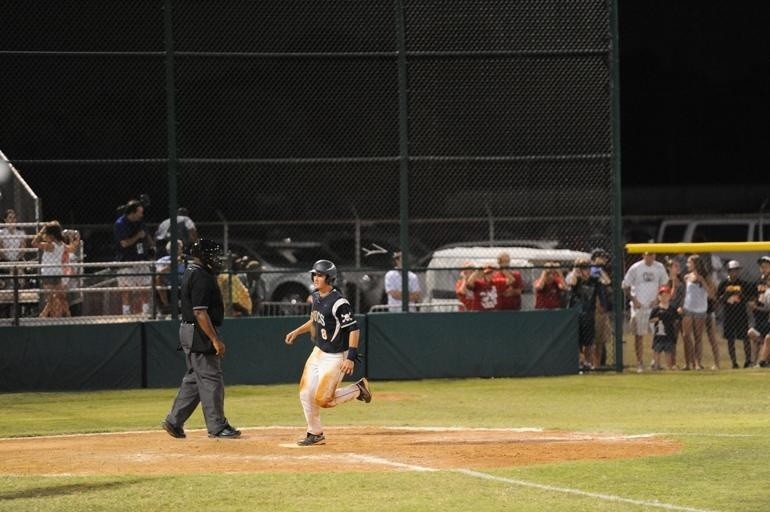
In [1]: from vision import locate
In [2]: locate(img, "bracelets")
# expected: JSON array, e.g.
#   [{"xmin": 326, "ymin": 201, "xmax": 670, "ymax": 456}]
[{"xmin": 347, "ymin": 348, "xmax": 359, "ymax": 362}]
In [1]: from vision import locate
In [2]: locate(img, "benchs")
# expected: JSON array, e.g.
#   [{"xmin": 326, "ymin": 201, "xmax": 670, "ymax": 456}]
[{"xmin": 1, "ymin": 222, "xmax": 84, "ymax": 306}]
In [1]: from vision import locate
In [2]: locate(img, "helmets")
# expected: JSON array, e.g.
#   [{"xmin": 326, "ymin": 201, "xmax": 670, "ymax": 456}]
[
  {"xmin": 191, "ymin": 240, "xmax": 222, "ymax": 275},
  {"xmin": 309, "ymin": 260, "xmax": 337, "ymax": 283}
]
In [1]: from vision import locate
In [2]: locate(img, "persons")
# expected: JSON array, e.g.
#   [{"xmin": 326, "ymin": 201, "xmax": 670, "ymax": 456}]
[
  {"xmin": 285, "ymin": 259, "xmax": 371, "ymax": 447},
  {"xmin": 0, "ymin": 199, "xmax": 262, "ymax": 317},
  {"xmin": 162, "ymin": 238, "xmax": 241, "ymax": 439},
  {"xmin": 384, "ymin": 251, "xmax": 420, "ymax": 312}
]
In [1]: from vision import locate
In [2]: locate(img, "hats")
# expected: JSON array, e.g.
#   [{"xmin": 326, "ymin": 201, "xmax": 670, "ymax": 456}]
[
  {"xmin": 757, "ymin": 255, "xmax": 770, "ymax": 265},
  {"xmin": 659, "ymin": 286, "xmax": 672, "ymax": 294},
  {"xmin": 590, "ymin": 249, "xmax": 609, "ymax": 261},
  {"xmin": 727, "ymin": 261, "xmax": 742, "ymax": 271}
]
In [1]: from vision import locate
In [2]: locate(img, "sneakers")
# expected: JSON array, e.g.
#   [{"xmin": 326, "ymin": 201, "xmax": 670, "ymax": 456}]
[
  {"xmin": 162, "ymin": 418, "xmax": 185, "ymax": 438},
  {"xmin": 208, "ymin": 425, "xmax": 241, "ymax": 438},
  {"xmin": 298, "ymin": 432, "xmax": 325, "ymax": 445},
  {"xmin": 357, "ymin": 377, "xmax": 371, "ymax": 404}
]
